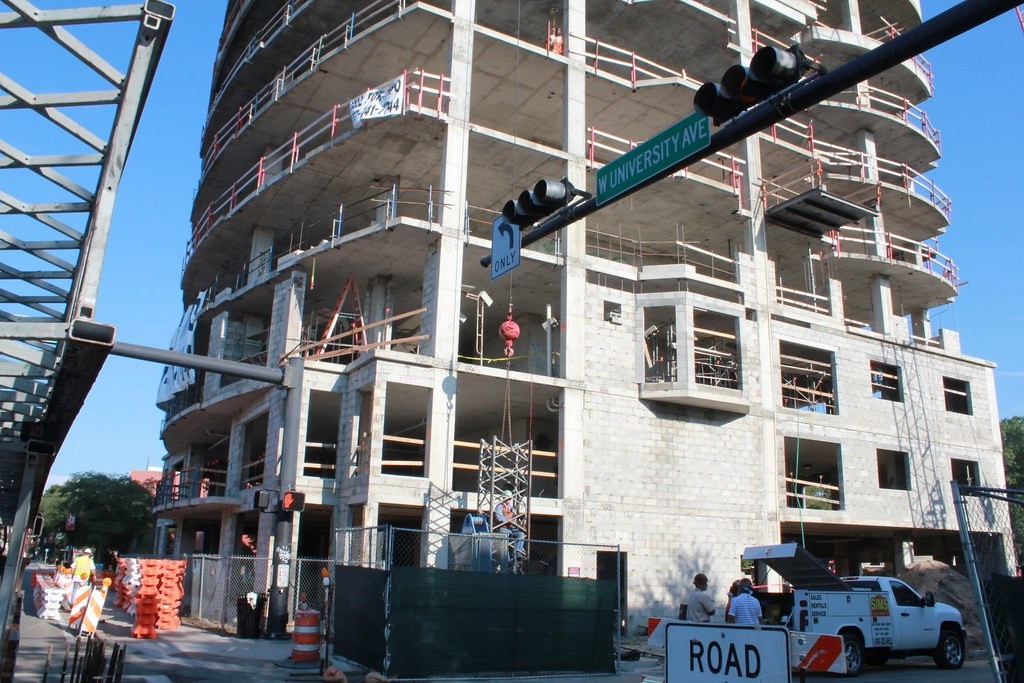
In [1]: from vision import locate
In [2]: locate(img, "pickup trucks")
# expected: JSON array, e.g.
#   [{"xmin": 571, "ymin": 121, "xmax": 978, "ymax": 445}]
[{"xmin": 741, "ymin": 543, "xmax": 968, "ymax": 675}]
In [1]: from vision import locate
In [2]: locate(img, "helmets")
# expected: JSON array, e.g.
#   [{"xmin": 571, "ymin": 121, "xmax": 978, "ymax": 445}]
[
  {"xmin": 731, "ymin": 579, "xmax": 741, "ymax": 586},
  {"xmin": 692, "ymin": 573, "xmax": 710, "ymax": 584},
  {"xmin": 736, "ymin": 578, "xmax": 752, "ymax": 588}
]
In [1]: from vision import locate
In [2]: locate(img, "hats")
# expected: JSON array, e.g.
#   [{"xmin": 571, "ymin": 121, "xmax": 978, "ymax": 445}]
[{"xmin": 84, "ymin": 548, "xmax": 93, "ymax": 555}]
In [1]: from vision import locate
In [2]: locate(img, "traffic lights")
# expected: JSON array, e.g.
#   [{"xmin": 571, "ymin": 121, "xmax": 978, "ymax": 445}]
[
  {"xmin": 502, "ymin": 179, "xmax": 566, "ymax": 226},
  {"xmin": 693, "ymin": 46, "xmax": 806, "ymax": 126},
  {"xmin": 282, "ymin": 491, "xmax": 305, "ymax": 512}
]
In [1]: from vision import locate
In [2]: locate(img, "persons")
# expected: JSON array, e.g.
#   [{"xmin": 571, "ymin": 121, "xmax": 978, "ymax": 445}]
[
  {"xmin": 685, "ymin": 574, "xmax": 715, "ymax": 623},
  {"xmin": 71, "ymin": 548, "xmax": 96, "ymax": 605},
  {"xmin": 495, "ymin": 490, "xmax": 526, "ymax": 556},
  {"xmin": 724, "ymin": 580, "xmax": 742, "ymax": 624},
  {"xmin": 727, "ymin": 578, "xmax": 764, "ymax": 625}
]
